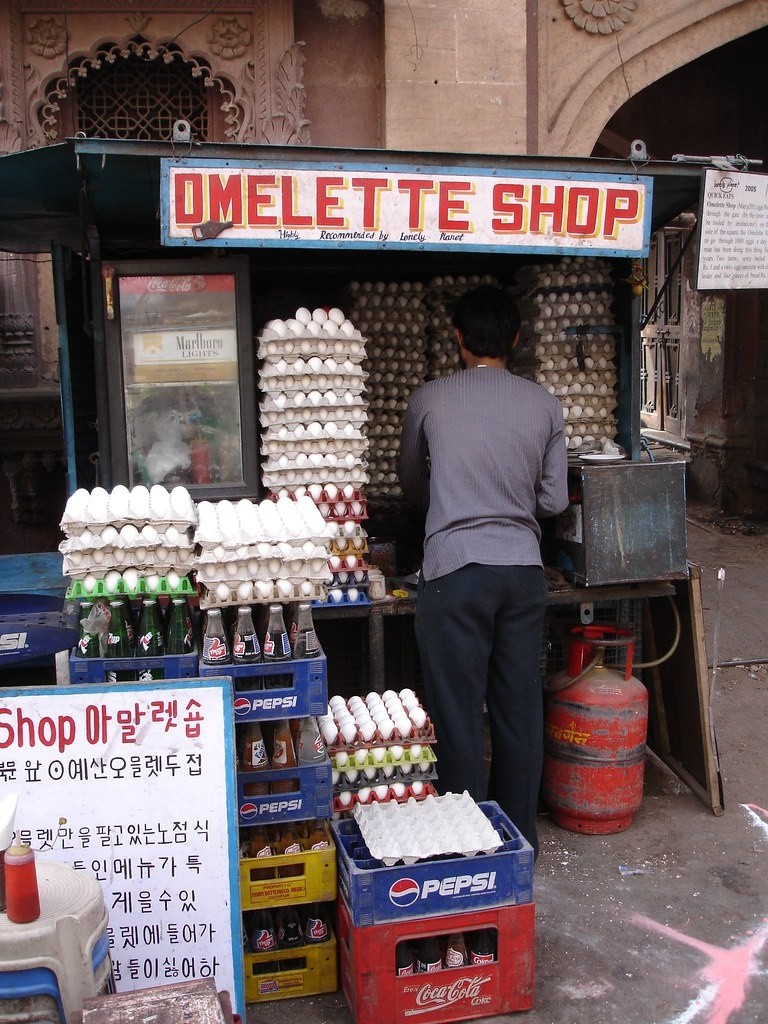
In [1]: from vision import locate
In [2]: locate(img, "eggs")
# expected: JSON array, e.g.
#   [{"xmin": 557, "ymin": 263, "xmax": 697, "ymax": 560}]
[
  {"xmin": 347, "ymin": 255, "xmax": 610, "ymax": 520},
  {"xmin": 263, "ymin": 305, "xmax": 364, "ymax": 603},
  {"xmin": 66, "ymin": 484, "xmax": 327, "ymax": 602},
  {"xmin": 317, "ymin": 688, "xmax": 429, "ymax": 805}
]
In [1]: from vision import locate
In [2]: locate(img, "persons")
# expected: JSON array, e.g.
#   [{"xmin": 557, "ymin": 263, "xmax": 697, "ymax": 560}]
[{"xmin": 395, "ymin": 284, "xmax": 568, "ymax": 867}]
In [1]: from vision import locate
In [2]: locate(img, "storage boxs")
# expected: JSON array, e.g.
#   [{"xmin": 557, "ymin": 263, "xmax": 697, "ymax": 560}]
[{"xmin": 68, "ymin": 595, "xmax": 643, "ymax": 1023}]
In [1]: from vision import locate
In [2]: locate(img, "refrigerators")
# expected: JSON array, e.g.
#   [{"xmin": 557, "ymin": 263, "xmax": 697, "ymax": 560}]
[{"xmin": 100, "ymin": 254, "xmax": 262, "ymax": 505}]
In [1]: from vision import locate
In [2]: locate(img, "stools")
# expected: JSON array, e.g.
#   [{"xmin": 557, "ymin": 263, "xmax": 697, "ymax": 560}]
[{"xmin": 1, "ymin": 856, "xmax": 225, "ymax": 1023}]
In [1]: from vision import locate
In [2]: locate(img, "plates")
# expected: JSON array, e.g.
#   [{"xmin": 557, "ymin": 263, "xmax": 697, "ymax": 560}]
[{"xmin": 578, "ymin": 454, "xmax": 626, "ymax": 467}]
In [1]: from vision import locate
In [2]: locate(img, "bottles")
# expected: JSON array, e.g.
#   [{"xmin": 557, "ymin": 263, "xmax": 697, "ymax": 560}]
[
  {"xmin": 201, "ymin": 601, "xmax": 323, "ymax": 690},
  {"xmin": 2, "ymin": 829, "xmax": 41, "ymax": 924},
  {"xmin": 73, "ymin": 597, "xmax": 197, "ymax": 683},
  {"xmin": 236, "ymin": 714, "xmax": 327, "ymax": 799},
  {"xmin": 242, "ymin": 903, "xmax": 330, "ymax": 976},
  {"xmin": 396, "ymin": 927, "xmax": 497, "ymax": 978},
  {"xmin": 247, "ymin": 819, "xmax": 330, "ymax": 882}
]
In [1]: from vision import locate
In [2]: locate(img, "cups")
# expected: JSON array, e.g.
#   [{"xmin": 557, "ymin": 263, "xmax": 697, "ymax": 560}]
[{"xmin": 368, "ymin": 563, "xmax": 386, "ymax": 599}]
